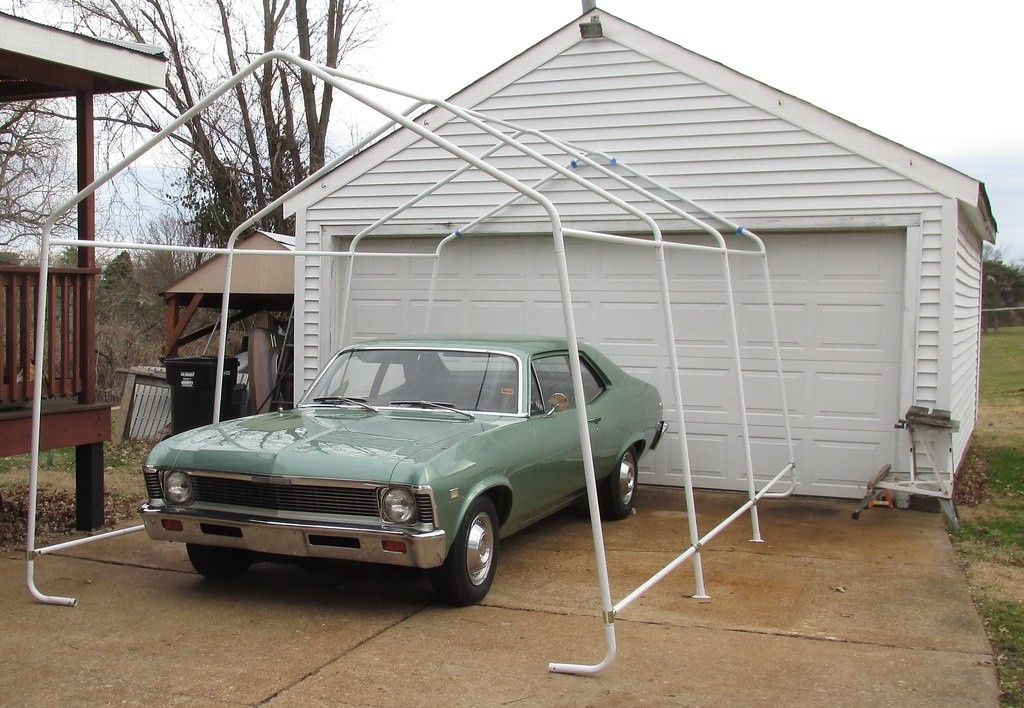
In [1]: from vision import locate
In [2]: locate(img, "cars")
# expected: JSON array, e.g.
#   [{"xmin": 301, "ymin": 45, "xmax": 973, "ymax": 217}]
[{"xmin": 135, "ymin": 334, "xmax": 669, "ymax": 606}]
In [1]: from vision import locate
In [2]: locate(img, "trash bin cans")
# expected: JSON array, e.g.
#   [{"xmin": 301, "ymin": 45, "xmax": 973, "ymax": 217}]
[{"xmin": 166, "ymin": 355, "xmax": 240, "ymax": 437}]
